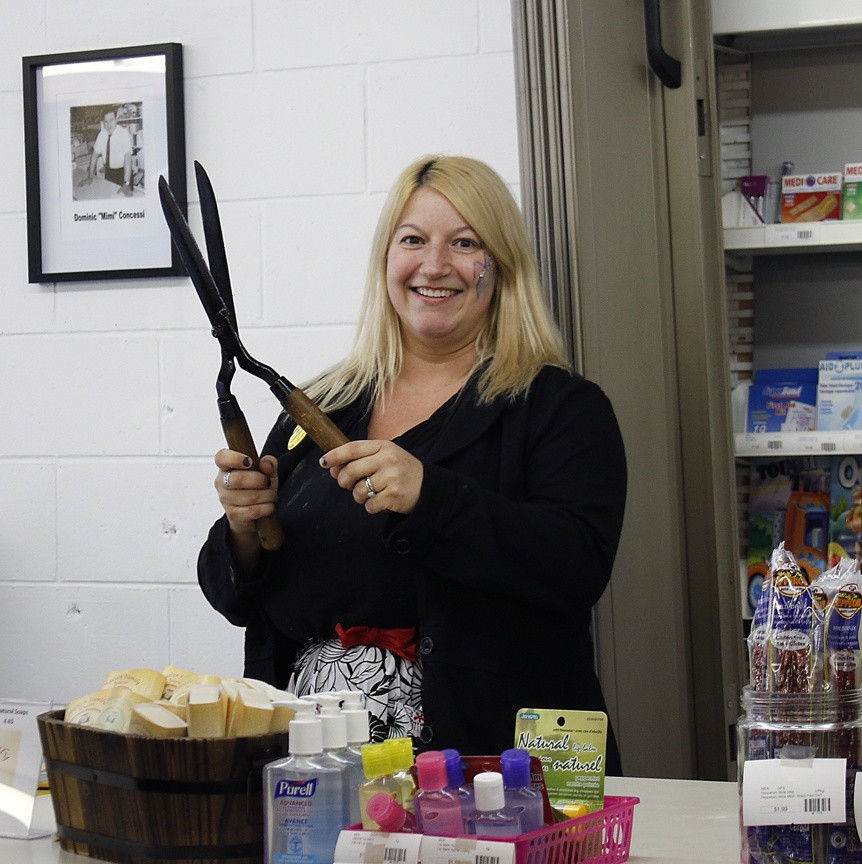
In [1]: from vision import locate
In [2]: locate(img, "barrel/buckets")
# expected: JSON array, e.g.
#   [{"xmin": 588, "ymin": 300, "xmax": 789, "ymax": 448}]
[{"xmin": 35, "ymin": 707, "xmax": 284, "ymax": 864}]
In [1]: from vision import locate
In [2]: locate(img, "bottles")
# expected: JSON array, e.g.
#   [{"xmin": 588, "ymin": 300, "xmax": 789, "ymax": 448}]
[
  {"xmin": 355, "ymin": 738, "xmax": 545, "ymax": 863},
  {"xmin": 735, "ymin": 683, "xmax": 862, "ymax": 864}
]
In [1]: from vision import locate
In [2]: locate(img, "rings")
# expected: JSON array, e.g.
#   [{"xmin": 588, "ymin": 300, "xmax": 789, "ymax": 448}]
[
  {"xmin": 365, "ymin": 476, "xmax": 375, "ymax": 498},
  {"xmin": 223, "ymin": 470, "xmax": 231, "ymax": 488}
]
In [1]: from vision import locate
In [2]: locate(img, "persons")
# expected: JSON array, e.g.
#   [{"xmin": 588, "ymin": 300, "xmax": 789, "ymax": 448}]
[
  {"xmin": 78, "ymin": 108, "xmax": 134, "ymax": 198},
  {"xmin": 197, "ymin": 155, "xmax": 627, "ymax": 778}
]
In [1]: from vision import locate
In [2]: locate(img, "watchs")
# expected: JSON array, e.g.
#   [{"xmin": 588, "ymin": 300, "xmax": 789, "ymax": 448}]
[{"xmin": 123, "ymin": 180, "xmax": 131, "ymax": 185}]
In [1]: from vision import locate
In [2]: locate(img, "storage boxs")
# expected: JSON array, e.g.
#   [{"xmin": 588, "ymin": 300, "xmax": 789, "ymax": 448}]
[
  {"xmin": 745, "ymin": 349, "xmax": 862, "ymax": 433},
  {"xmin": 779, "ymin": 163, "xmax": 861, "ymax": 223}
]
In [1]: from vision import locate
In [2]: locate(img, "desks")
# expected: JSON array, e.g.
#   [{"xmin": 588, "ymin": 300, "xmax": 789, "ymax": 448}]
[{"xmin": 1, "ymin": 773, "xmax": 743, "ymax": 864}]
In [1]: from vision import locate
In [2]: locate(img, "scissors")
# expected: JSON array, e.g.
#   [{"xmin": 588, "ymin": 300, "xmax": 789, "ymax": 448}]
[{"xmin": 158, "ymin": 160, "xmax": 357, "ymax": 552}]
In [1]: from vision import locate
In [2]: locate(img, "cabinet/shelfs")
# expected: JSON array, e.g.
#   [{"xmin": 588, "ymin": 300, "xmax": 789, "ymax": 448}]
[{"xmin": 707, "ymin": 1, "xmax": 862, "ymax": 620}]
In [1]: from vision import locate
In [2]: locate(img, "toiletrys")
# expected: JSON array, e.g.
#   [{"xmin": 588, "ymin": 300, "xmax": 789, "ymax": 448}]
[
  {"xmin": 358, "ymin": 736, "xmax": 544, "ymax": 836},
  {"xmin": 260, "ymin": 688, "xmax": 371, "ymax": 861}
]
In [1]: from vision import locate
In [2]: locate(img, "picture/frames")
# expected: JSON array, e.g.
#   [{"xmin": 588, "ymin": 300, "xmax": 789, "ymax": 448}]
[{"xmin": 20, "ymin": 42, "xmax": 187, "ymax": 284}]
